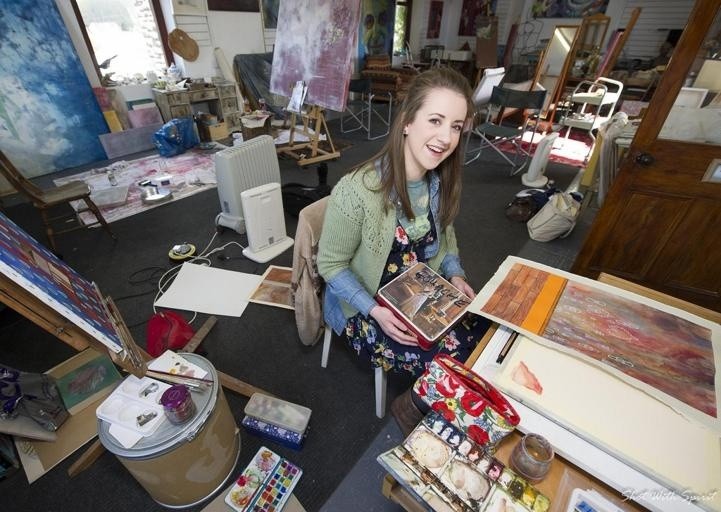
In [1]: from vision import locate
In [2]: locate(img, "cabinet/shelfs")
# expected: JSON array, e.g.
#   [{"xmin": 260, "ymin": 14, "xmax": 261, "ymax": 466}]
[
  {"xmin": 558, "ymin": 77, "xmax": 623, "ymax": 165},
  {"xmin": 151, "ymin": 81, "xmax": 242, "ymax": 132}
]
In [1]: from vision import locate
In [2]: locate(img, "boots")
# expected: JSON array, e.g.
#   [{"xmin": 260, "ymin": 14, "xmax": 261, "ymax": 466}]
[{"xmin": 390, "ymin": 385, "xmax": 424, "ymax": 439}]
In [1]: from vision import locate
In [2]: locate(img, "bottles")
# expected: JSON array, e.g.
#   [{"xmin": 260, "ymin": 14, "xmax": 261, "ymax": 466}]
[
  {"xmin": 509, "ymin": 433, "xmax": 555, "ymax": 485},
  {"xmin": 161, "ymin": 386, "xmax": 195, "ymax": 426},
  {"xmin": 108, "ymin": 171, "xmax": 117, "ymax": 186}
]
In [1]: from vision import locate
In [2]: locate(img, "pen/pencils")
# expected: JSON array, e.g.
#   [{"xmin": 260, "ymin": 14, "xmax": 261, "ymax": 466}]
[{"xmin": 496, "ymin": 332, "xmax": 518, "ymax": 364}]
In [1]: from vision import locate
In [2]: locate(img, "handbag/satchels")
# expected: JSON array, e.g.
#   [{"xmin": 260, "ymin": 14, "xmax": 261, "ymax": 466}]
[
  {"xmin": 413, "ymin": 353, "xmax": 520, "ymax": 455},
  {"xmin": 526, "ymin": 192, "xmax": 580, "ymax": 243}
]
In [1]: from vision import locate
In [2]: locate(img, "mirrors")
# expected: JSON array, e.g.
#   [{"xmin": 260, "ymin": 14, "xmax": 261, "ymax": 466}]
[
  {"xmin": 567, "ymin": 12, "xmax": 611, "ymax": 77},
  {"xmin": 523, "ymin": 24, "xmax": 582, "ymax": 135}
]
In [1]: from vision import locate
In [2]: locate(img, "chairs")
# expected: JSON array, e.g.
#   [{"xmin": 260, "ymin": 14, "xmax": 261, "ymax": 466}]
[
  {"xmin": 359, "ymin": 55, "xmax": 418, "ymax": 107},
  {"xmin": 297, "ymin": 195, "xmax": 387, "ymax": 419},
  {"xmin": 340, "ymin": 76, "xmax": 392, "ymax": 141},
  {"xmin": 0, "ymin": 149, "xmax": 117, "ymax": 255},
  {"xmin": 620, "ymin": 76, "xmax": 655, "ymax": 101},
  {"xmin": 463, "ymin": 86, "xmax": 547, "ymax": 178}
]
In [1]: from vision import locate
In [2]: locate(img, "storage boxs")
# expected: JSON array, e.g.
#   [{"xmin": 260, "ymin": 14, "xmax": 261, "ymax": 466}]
[{"xmin": 198, "ymin": 124, "xmax": 228, "ymax": 140}]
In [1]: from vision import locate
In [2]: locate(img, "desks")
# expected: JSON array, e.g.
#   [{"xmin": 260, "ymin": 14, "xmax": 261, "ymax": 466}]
[
  {"xmin": 432, "ymin": 59, "xmax": 476, "ymax": 88},
  {"xmin": 380, "ymin": 267, "xmax": 721, "ymax": 511},
  {"xmin": 578, "ymin": 127, "xmax": 633, "ymax": 220}
]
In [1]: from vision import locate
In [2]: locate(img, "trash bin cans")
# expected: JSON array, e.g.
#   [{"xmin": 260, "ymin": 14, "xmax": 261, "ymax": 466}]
[{"xmin": 240, "ymin": 110, "xmax": 272, "ymax": 142}]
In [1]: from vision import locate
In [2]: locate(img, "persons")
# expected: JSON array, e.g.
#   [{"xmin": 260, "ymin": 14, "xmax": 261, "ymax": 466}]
[
  {"xmin": 363, "ymin": 9, "xmax": 389, "ymax": 55},
  {"xmin": 460, "ymin": 8, "xmax": 470, "ymax": 37},
  {"xmin": 316, "ymin": 68, "xmax": 489, "ymax": 438}
]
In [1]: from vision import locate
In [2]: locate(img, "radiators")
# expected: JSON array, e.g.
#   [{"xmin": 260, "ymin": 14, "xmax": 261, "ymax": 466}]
[{"xmin": 214, "ymin": 133, "xmax": 281, "ymax": 234}]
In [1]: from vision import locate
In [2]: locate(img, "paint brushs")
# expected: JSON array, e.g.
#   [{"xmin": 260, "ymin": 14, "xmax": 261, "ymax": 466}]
[{"xmin": 145, "ymin": 370, "xmax": 215, "ymax": 393}]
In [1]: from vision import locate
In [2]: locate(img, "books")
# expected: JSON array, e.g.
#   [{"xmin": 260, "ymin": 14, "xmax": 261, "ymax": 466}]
[{"xmin": 54, "ymin": 352, "xmax": 122, "ymax": 416}]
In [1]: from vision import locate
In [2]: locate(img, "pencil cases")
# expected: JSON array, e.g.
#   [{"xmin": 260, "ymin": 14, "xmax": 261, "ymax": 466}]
[{"xmin": 241, "ymin": 392, "xmax": 313, "ymax": 451}]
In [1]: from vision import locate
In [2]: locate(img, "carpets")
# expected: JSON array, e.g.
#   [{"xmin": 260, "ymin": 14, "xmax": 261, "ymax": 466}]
[
  {"xmin": 485, "ymin": 119, "xmax": 595, "ymax": 167},
  {"xmin": 51, "ymin": 141, "xmax": 232, "ymax": 229}
]
